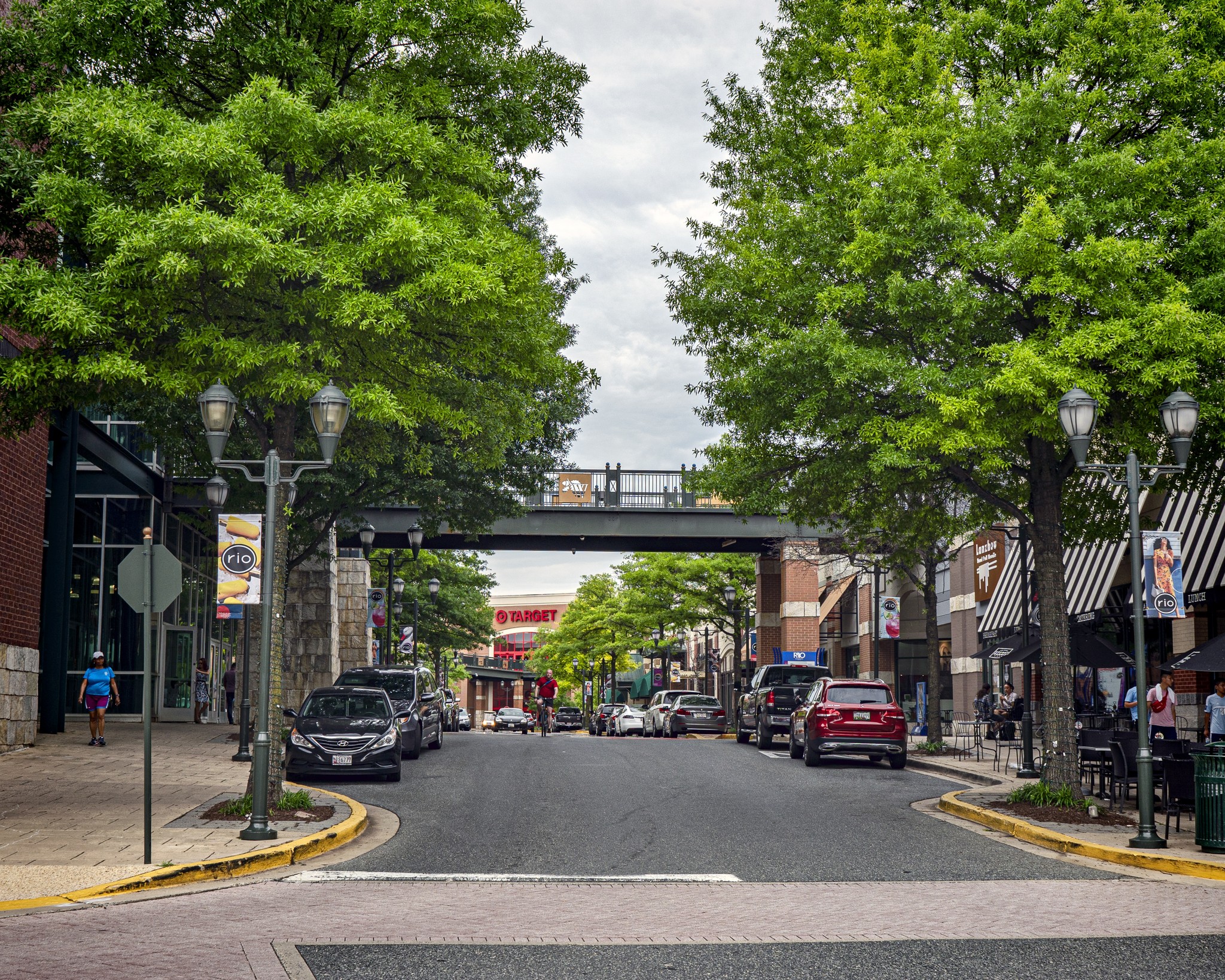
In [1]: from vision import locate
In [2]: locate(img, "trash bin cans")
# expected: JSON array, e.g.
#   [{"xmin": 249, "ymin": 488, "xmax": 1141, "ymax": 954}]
[{"xmin": 1191, "ymin": 752, "xmax": 1225, "ymax": 854}]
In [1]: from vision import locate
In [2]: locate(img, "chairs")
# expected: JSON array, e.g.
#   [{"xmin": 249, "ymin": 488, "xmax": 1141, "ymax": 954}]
[
  {"xmin": 318, "ymin": 698, "xmax": 338, "ymax": 716},
  {"xmin": 801, "ymin": 676, "xmax": 816, "ymax": 684},
  {"xmin": 339, "ymin": 700, "xmax": 357, "ymax": 716},
  {"xmin": 353, "ymin": 698, "xmax": 385, "ymax": 717},
  {"xmin": 991, "ymin": 703, "xmax": 1225, "ymax": 841},
  {"xmin": 949, "ymin": 711, "xmax": 984, "ymax": 760},
  {"xmin": 918, "ymin": 703, "xmax": 928, "ymax": 737}
]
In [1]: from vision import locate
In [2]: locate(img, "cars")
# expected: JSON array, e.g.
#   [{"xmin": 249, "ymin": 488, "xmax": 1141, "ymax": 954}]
[
  {"xmin": 588, "ymin": 703, "xmax": 626, "ymax": 736},
  {"xmin": 614, "ymin": 704, "xmax": 649, "ymax": 737},
  {"xmin": 604, "ymin": 706, "xmax": 624, "ymax": 736},
  {"xmin": 662, "ymin": 693, "xmax": 728, "ymax": 738},
  {"xmin": 437, "ymin": 687, "xmax": 584, "ymax": 734},
  {"xmin": 283, "ymin": 685, "xmax": 411, "ymax": 782},
  {"xmin": 641, "ymin": 689, "xmax": 704, "ymax": 738},
  {"xmin": 331, "ymin": 664, "xmax": 445, "ymax": 760}
]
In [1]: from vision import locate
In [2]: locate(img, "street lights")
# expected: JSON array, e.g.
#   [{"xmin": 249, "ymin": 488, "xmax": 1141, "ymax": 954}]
[
  {"xmin": 572, "ymin": 658, "xmax": 595, "ymax": 731},
  {"xmin": 194, "ymin": 375, "xmax": 353, "ymax": 843},
  {"xmin": 982, "ymin": 496, "xmax": 1041, "ymax": 780},
  {"xmin": 724, "ymin": 585, "xmax": 757, "ymax": 685},
  {"xmin": 392, "ymin": 574, "xmax": 441, "ymax": 668},
  {"xmin": 358, "ymin": 521, "xmax": 425, "ymax": 664},
  {"xmin": 845, "ymin": 529, "xmax": 894, "ymax": 680},
  {"xmin": 1056, "ymin": 385, "xmax": 1201, "ymax": 850},
  {"xmin": 651, "ymin": 629, "xmax": 687, "ymax": 691},
  {"xmin": 689, "ymin": 619, "xmax": 725, "ymax": 696}
]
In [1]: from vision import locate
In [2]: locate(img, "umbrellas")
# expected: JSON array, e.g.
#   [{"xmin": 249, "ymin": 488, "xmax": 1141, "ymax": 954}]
[
  {"xmin": 969, "ymin": 620, "xmax": 1134, "ymax": 714},
  {"xmin": 1154, "ymin": 632, "xmax": 1225, "ymax": 672}
]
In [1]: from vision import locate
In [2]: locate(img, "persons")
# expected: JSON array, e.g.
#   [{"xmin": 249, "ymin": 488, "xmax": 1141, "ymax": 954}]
[
  {"xmin": 78, "ymin": 651, "xmax": 121, "ymax": 746},
  {"xmin": 1124, "ymin": 670, "xmax": 1178, "ymax": 743},
  {"xmin": 1203, "ymin": 677, "xmax": 1225, "ymax": 743},
  {"xmin": 168, "ymin": 677, "xmax": 186, "ymax": 708},
  {"xmin": 973, "ymin": 682, "xmax": 1018, "ymax": 740},
  {"xmin": 196, "ymin": 658, "xmax": 210, "ymax": 724},
  {"xmin": 223, "ymin": 662, "xmax": 236, "ymax": 725},
  {"xmin": 534, "ymin": 669, "xmax": 559, "ymax": 733}
]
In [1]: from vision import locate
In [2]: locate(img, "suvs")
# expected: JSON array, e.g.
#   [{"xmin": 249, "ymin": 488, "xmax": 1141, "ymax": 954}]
[
  {"xmin": 789, "ymin": 676, "xmax": 908, "ymax": 769},
  {"xmin": 735, "ymin": 664, "xmax": 834, "ymax": 750}
]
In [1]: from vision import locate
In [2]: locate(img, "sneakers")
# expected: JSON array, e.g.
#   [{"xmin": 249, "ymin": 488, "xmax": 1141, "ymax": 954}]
[
  {"xmin": 88, "ymin": 737, "xmax": 98, "ymax": 746},
  {"xmin": 98, "ymin": 736, "xmax": 107, "ymax": 746}
]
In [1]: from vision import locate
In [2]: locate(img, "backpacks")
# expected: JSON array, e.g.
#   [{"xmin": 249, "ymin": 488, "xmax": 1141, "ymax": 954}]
[
  {"xmin": 1009, "ymin": 695, "xmax": 1024, "ymax": 721},
  {"xmin": 1000, "ymin": 721, "xmax": 1016, "ymax": 741}
]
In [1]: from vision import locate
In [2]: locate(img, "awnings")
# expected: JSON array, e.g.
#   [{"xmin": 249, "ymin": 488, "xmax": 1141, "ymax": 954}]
[
  {"xmin": 978, "ymin": 434, "xmax": 1225, "ymax": 636},
  {"xmin": 818, "ymin": 574, "xmax": 856, "ymax": 626},
  {"xmin": 605, "ymin": 666, "xmax": 663, "ymax": 704},
  {"xmin": 680, "ymin": 671, "xmax": 705, "ymax": 679}
]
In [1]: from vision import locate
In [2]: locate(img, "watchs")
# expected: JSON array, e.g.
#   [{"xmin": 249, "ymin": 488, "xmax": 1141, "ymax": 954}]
[{"xmin": 116, "ymin": 694, "xmax": 120, "ymax": 697}]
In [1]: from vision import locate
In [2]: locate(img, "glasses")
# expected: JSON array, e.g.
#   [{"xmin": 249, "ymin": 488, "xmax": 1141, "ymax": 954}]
[
  {"xmin": 198, "ymin": 662, "xmax": 202, "ymax": 664},
  {"xmin": 1160, "ymin": 537, "xmax": 1168, "ymax": 541}
]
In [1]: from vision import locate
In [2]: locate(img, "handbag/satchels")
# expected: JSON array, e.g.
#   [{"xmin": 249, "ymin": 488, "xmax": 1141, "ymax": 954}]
[
  {"xmin": 1150, "ymin": 690, "xmax": 1168, "ymax": 713},
  {"xmin": 109, "ymin": 695, "xmax": 113, "ymax": 708},
  {"xmin": 1150, "ymin": 584, "xmax": 1164, "ymax": 604}
]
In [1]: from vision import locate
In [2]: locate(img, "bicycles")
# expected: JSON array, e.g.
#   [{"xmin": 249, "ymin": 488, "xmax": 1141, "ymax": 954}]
[{"xmin": 532, "ymin": 696, "xmax": 554, "ymax": 737}]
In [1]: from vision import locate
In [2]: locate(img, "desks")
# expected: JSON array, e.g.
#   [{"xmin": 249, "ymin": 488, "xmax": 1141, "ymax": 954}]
[
  {"xmin": 904, "ymin": 710, "xmax": 913, "ymax": 743},
  {"xmin": 957, "ymin": 720, "xmax": 999, "ymax": 763},
  {"xmin": 1076, "ymin": 712, "xmax": 1108, "ymax": 730},
  {"xmin": 1178, "ymin": 727, "xmax": 1209, "ymax": 743},
  {"xmin": 995, "ymin": 707, "xmax": 1010, "ymax": 719},
  {"xmin": 1096, "ymin": 714, "xmax": 1132, "ymax": 741},
  {"xmin": 1031, "ymin": 726, "xmax": 1044, "ymax": 773},
  {"xmin": 1077, "ymin": 744, "xmax": 1112, "ymax": 800},
  {"xmin": 1150, "ymin": 754, "xmax": 1181, "ymax": 816},
  {"xmin": 940, "ymin": 709, "xmax": 953, "ymax": 737}
]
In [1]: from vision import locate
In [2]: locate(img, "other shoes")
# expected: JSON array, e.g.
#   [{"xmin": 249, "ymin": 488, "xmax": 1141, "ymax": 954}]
[
  {"xmin": 229, "ymin": 723, "xmax": 233, "ymax": 725},
  {"xmin": 195, "ymin": 719, "xmax": 203, "ymax": 724},
  {"xmin": 986, "ymin": 730, "xmax": 995, "ymax": 738},
  {"xmin": 548, "ymin": 728, "xmax": 551, "ymax": 732},
  {"xmin": 538, "ymin": 716, "xmax": 541, "ymax": 721},
  {"xmin": 199, "ymin": 714, "xmax": 201, "ymax": 719},
  {"xmin": 985, "ymin": 735, "xmax": 994, "ymax": 740}
]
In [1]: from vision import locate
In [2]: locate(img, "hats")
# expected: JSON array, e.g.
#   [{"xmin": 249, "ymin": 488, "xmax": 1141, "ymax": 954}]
[{"xmin": 93, "ymin": 651, "xmax": 104, "ymax": 658}]
